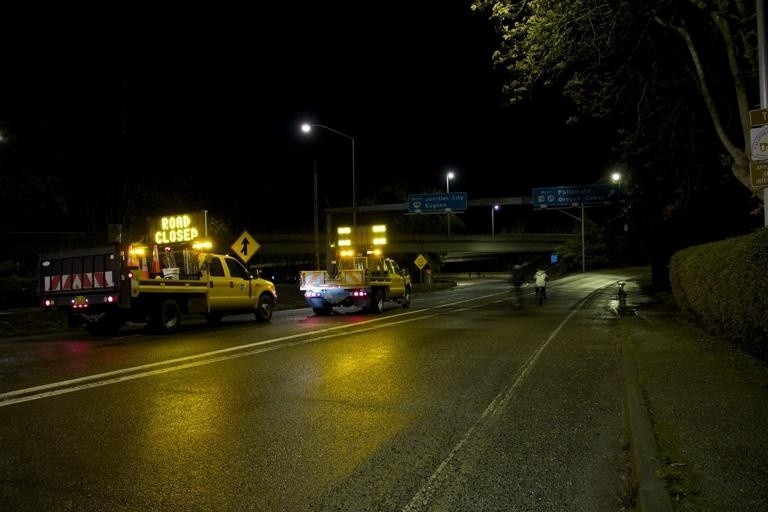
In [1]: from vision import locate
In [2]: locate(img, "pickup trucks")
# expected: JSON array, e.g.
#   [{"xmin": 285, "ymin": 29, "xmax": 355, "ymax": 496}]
[
  {"xmin": 299, "ymin": 254, "xmax": 411, "ymax": 316},
  {"xmin": 39, "ymin": 241, "xmax": 276, "ymax": 337}
]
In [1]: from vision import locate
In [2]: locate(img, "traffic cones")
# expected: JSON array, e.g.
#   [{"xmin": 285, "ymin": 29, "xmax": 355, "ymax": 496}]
[
  {"xmin": 138, "ymin": 254, "xmax": 149, "ymax": 279},
  {"xmin": 126, "ymin": 245, "xmax": 136, "ymax": 266},
  {"xmin": 149, "ymin": 244, "xmax": 162, "ymax": 274}
]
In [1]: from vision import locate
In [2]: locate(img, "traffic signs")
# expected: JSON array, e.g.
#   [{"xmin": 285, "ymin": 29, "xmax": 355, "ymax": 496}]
[
  {"xmin": 408, "ymin": 192, "xmax": 468, "ymax": 211},
  {"xmin": 533, "ymin": 183, "xmax": 619, "ymax": 207}
]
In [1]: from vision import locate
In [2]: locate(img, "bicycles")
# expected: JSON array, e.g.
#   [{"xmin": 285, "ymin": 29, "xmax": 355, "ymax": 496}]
[
  {"xmin": 616, "ymin": 279, "xmax": 629, "ymax": 318},
  {"xmin": 533, "ymin": 278, "xmax": 549, "ymax": 306},
  {"xmin": 510, "ymin": 278, "xmax": 527, "ymax": 310}
]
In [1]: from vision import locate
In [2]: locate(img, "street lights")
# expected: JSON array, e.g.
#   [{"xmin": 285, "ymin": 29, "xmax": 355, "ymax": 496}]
[
  {"xmin": 299, "ymin": 123, "xmax": 359, "ymax": 259},
  {"xmin": 447, "ymin": 171, "xmax": 454, "ymax": 235},
  {"xmin": 491, "ymin": 205, "xmax": 500, "ymax": 237}
]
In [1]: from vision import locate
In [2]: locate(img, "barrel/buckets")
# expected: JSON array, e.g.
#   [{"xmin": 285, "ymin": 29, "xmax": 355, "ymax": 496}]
[{"xmin": 162, "ymin": 266, "xmax": 180, "ymax": 279}]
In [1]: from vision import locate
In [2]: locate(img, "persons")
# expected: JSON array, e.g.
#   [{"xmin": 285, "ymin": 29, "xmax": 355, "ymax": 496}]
[
  {"xmin": 510, "ymin": 265, "xmax": 525, "ymax": 309},
  {"xmin": 424, "ymin": 256, "xmax": 433, "ymax": 288},
  {"xmin": 532, "ymin": 265, "xmax": 549, "ymax": 301}
]
